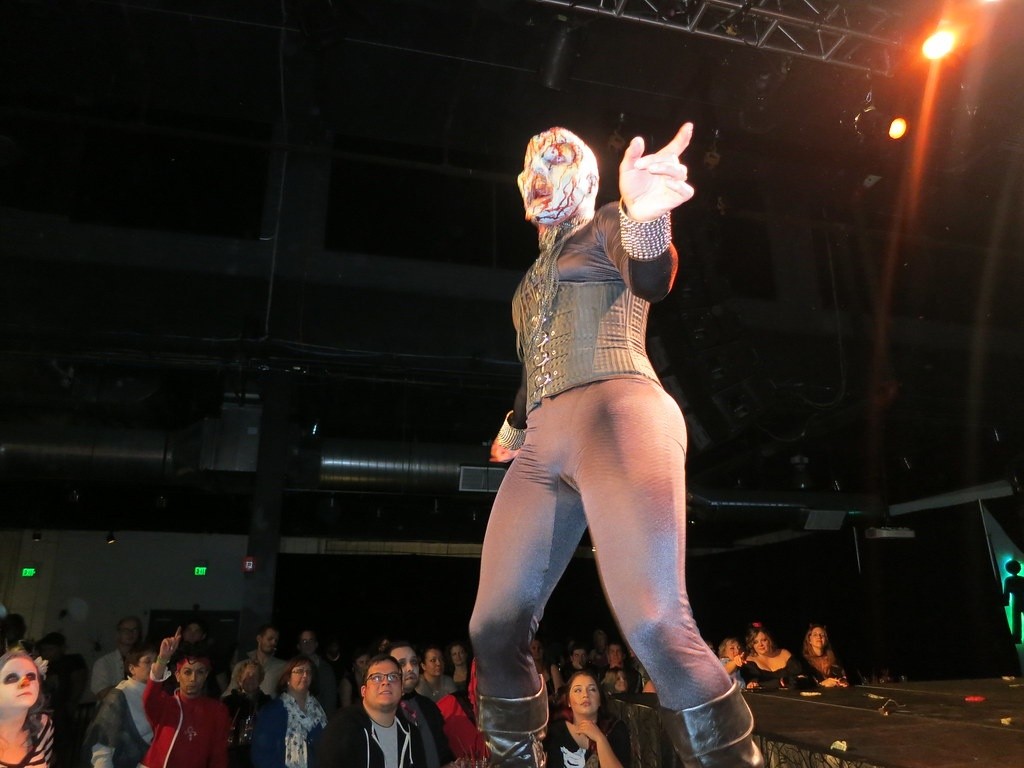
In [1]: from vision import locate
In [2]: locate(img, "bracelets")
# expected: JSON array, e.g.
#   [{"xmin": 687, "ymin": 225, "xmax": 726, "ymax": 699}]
[
  {"xmin": 497, "ymin": 411, "xmax": 526, "ymax": 450},
  {"xmin": 157, "ymin": 656, "xmax": 171, "ymax": 665},
  {"xmin": 618, "ymin": 199, "xmax": 673, "ymax": 259}
]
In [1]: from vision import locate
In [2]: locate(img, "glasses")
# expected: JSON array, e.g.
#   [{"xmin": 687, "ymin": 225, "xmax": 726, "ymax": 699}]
[
  {"xmin": 364, "ymin": 672, "xmax": 402, "ymax": 684},
  {"xmin": 301, "ymin": 639, "xmax": 316, "ymax": 646},
  {"xmin": 121, "ymin": 628, "xmax": 140, "ymax": 633},
  {"xmin": 290, "ymin": 669, "xmax": 312, "ymax": 677}
]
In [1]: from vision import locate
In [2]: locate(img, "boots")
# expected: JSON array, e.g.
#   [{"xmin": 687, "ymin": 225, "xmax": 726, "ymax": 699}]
[
  {"xmin": 478, "ymin": 673, "xmax": 549, "ymax": 768},
  {"xmin": 663, "ymin": 678, "xmax": 764, "ymax": 768}
]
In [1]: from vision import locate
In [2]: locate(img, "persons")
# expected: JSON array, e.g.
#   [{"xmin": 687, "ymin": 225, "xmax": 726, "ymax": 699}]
[
  {"xmin": 0, "ymin": 613, "xmax": 90, "ymax": 768},
  {"xmin": 81, "ymin": 617, "xmax": 489, "ymax": 768},
  {"xmin": 530, "ymin": 629, "xmax": 657, "ymax": 768},
  {"xmin": 702, "ymin": 621, "xmax": 849, "ymax": 692},
  {"xmin": 468, "ymin": 124, "xmax": 767, "ymax": 768}
]
